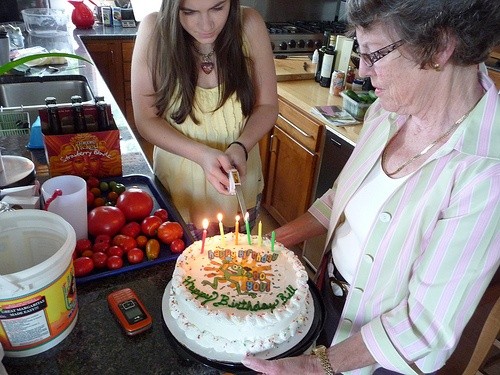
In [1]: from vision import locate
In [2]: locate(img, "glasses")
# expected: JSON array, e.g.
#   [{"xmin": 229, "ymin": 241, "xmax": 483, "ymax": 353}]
[{"xmin": 351, "ymin": 36, "xmax": 410, "ymax": 68}]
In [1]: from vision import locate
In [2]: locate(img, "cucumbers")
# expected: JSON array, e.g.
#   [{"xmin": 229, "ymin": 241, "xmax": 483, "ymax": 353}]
[{"xmin": 344, "ymin": 90, "xmax": 378, "ymax": 122}]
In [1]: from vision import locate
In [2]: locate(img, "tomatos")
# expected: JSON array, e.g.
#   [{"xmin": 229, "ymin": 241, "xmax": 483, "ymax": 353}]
[{"xmin": 73, "ymin": 176, "xmax": 185, "ymax": 276}]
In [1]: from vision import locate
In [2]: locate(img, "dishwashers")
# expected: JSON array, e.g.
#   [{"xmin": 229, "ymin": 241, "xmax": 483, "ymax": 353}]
[{"xmin": 302, "ymin": 125, "xmax": 357, "ymax": 273}]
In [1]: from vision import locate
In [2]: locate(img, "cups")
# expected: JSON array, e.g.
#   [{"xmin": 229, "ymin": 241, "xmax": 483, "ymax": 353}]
[{"xmin": 40, "ymin": 175, "xmax": 88, "ymax": 240}]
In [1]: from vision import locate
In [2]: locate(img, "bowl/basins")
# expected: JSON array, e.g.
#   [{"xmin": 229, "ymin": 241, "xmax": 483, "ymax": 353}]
[
  {"xmin": 20, "ymin": 8, "xmax": 71, "ymax": 38},
  {"xmin": 94, "ymin": 6, "xmax": 133, "ymax": 24}
]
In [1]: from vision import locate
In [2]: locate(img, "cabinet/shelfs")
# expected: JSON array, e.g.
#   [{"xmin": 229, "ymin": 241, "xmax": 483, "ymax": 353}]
[
  {"xmin": 84, "ymin": 40, "xmax": 143, "ymax": 139},
  {"xmin": 259, "ymin": 95, "xmax": 326, "ymax": 251}
]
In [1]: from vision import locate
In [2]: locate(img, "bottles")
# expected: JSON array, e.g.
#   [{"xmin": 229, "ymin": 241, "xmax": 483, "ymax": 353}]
[
  {"xmin": 0, "ymin": 31, "xmax": 10, "ymax": 67},
  {"xmin": 71, "ymin": 95, "xmax": 88, "ymax": 133},
  {"xmin": 102, "ymin": 7, "xmax": 111, "ymax": 26},
  {"xmin": 319, "ymin": 34, "xmax": 337, "ymax": 87},
  {"xmin": 45, "ymin": 96, "xmax": 63, "ymax": 135},
  {"xmin": 345, "ymin": 66, "xmax": 373, "ymax": 92},
  {"xmin": 312, "ymin": 48, "xmax": 319, "ymax": 77},
  {"xmin": 94, "ymin": 94, "xmax": 111, "ymax": 131},
  {"xmin": 112, "ymin": 7, "xmax": 121, "ymax": 27},
  {"xmin": 316, "ymin": 31, "xmax": 330, "ymax": 82}
]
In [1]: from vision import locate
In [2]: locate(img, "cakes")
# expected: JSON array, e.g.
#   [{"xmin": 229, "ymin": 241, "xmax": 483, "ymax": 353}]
[{"xmin": 170, "ymin": 232, "xmax": 310, "ymax": 354}]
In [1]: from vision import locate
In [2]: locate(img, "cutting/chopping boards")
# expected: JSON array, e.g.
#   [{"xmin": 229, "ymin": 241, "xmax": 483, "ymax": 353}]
[{"xmin": 273, "ymin": 57, "xmax": 317, "ymax": 81}]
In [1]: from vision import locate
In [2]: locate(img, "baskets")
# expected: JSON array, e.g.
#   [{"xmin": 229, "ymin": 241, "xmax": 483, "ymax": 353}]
[{"xmin": 339, "ymin": 89, "xmax": 378, "ymax": 121}]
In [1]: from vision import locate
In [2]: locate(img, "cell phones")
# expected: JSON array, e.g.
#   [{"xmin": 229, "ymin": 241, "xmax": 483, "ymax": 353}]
[{"xmin": 107, "ymin": 288, "xmax": 154, "ymax": 336}]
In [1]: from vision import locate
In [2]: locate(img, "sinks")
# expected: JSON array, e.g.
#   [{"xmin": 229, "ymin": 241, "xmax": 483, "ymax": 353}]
[{"xmin": 0, "ymin": 80, "xmax": 96, "ymax": 111}]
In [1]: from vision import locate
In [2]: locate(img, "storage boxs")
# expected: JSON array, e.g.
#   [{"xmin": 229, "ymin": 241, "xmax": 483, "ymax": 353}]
[
  {"xmin": 339, "ymin": 91, "xmax": 370, "ymax": 121},
  {"xmin": 38, "ymin": 104, "xmax": 123, "ymax": 178}
]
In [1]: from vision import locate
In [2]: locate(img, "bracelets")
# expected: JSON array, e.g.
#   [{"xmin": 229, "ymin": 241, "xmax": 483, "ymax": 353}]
[{"xmin": 226, "ymin": 141, "xmax": 248, "ymax": 161}]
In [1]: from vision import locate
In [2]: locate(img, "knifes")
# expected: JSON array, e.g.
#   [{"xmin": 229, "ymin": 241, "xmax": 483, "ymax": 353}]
[{"xmin": 228, "ymin": 169, "xmax": 248, "ymax": 218}]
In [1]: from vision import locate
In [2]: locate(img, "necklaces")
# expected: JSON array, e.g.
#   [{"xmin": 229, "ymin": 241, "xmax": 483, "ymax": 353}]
[
  {"xmin": 191, "ymin": 41, "xmax": 217, "ymax": 75},
  {"xmin": 381, "ymin": 86, "xmax": 485, "ymax": 176}
]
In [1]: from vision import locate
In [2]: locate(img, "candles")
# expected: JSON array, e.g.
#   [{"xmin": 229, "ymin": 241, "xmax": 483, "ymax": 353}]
[{"xmin": 201, "ymin": 212, "xmax": 275, "ymax": 254}]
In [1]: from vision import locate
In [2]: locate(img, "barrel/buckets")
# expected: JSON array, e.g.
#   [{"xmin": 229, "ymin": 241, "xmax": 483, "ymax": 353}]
[{"xmin": 0, "ymin": 209, "xmax": 79, "ymax": 357}]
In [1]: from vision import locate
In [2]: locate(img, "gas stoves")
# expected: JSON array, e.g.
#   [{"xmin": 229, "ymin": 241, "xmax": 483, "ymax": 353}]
[{"xmin": 265, "ymin": 19, "xmax": 357, "ymax": 58}]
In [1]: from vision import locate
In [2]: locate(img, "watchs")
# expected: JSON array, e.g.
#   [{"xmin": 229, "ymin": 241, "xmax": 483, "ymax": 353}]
[{"xmin": 309, "ymin": 344, "xmax": 334, "ymax": 375}]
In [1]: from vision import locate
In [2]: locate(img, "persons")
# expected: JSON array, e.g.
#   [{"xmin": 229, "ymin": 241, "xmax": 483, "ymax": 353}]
[
  {"xmin": 241, "ymin": 0, "xmax": 500, "ymax": 375},
  {"xmin": 131, "ymin": 0, "xmax": 280, "ymax": 242}
]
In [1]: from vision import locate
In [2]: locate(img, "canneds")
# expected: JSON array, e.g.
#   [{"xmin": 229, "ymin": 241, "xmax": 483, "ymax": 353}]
[
  {"xmin": 329, "ymin": 69, "xmax": 345, "ymax": 96},
  {"xmin": 101, "ymin": 7, "xmax": 122, "ymax": 27}
]
